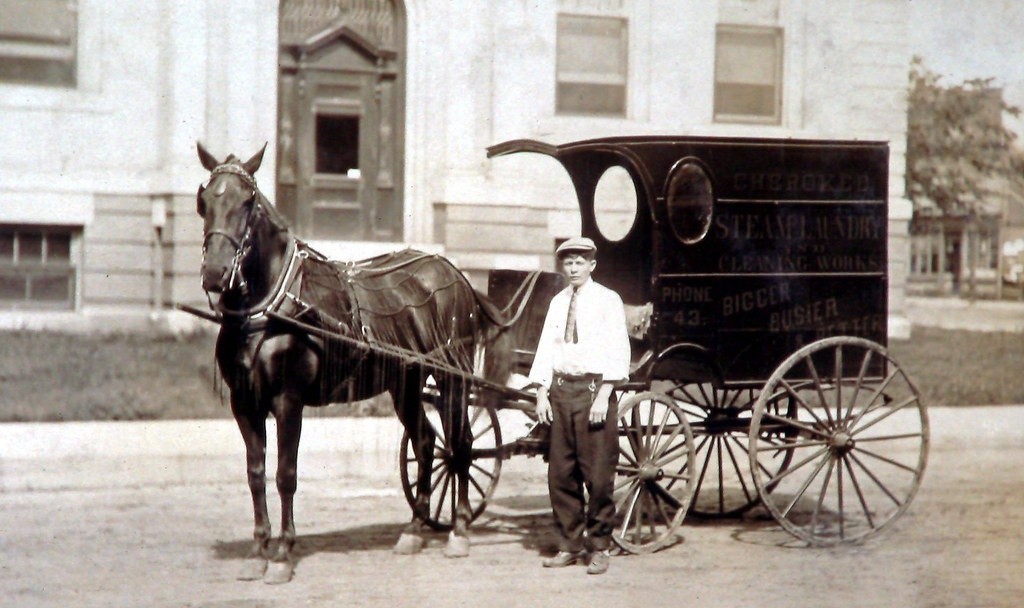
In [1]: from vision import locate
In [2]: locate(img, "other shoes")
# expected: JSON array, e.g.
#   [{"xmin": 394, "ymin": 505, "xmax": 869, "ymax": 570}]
[
  {"xmin": 586, "ymin": 551, "xmax": 610, "ymax": 573},
  {"xmin": 543, "ymin": 552, "xmax": 577, "ymax": 568}
]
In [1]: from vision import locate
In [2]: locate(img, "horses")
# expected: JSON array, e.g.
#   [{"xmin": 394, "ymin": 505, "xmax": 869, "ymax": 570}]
[{"xmin": 195, "ymin": 143, "xmax": 501, "ymax": 585}]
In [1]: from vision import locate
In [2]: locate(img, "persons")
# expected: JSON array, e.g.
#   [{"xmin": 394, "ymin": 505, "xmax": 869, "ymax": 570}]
[{"xmin": 527, "ymin": 235, "xmax": 631, "ymax": 574}]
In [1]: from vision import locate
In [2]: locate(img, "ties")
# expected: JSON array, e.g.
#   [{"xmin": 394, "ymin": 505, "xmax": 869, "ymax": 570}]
[{"xmin": 564, "ymin": 287, "xmax": 578, "ymax": 343}]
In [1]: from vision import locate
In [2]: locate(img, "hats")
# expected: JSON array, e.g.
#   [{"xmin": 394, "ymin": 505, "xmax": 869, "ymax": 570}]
[{"xmin": 556, "ymin": 237, "xmax": 598, "ymax": 258}]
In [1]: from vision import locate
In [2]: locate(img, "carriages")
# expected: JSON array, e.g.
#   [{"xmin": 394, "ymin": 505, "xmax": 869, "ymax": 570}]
[{"xmin": 159, "ymin": 131, "xmax": 932, "ymax": 586}]
